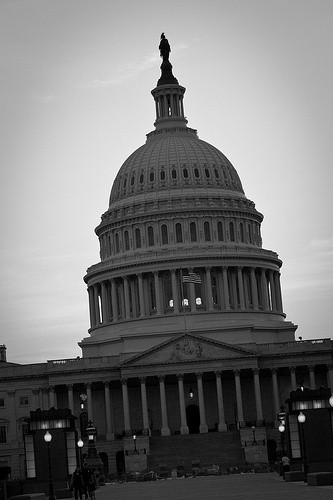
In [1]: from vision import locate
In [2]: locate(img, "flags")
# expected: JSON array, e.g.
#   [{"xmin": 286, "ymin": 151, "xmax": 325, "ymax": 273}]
[{"xmin": 182, "ymin": 271, "xmax": 202, "ymax": 283}]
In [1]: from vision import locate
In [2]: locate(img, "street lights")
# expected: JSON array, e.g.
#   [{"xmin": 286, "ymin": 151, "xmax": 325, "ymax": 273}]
[
  {"xmin": 298, "ymin": 412, "xmax": 308, "ymax": 483},
  {"xmin": 77, "ymin": 438, "xmax": 84, "ymax": 500},
  {"xmin": 43, "ymin": 430, "xmax": 55, "ymax": 500},
  {"xmin": 278, "ymin": 424, "xmax": 286, "ymax": 480}
]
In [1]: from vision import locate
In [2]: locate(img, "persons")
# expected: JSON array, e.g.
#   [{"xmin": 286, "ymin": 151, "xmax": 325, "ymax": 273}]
[
  {"xmin": 70, "ymin": 468, "xmax": 83, "ymax": 500},
  {"xmin": 280, "ymin": 454, "xmax": 292, "ymax": 480},
  {"xmin": 81, "ymin": 468, "xmax": 96, "ymax": 500}
]
